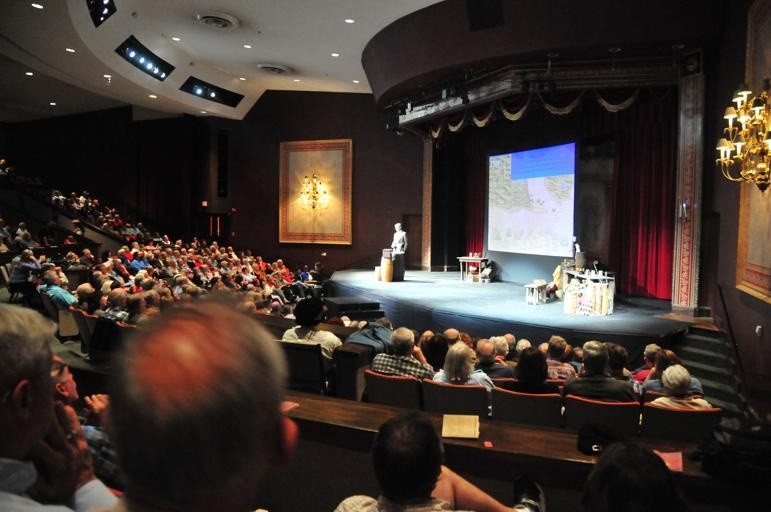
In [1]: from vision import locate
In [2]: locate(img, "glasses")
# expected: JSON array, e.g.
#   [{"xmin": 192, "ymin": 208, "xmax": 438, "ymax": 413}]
[{"xmin": 51, "ymin": 360, "xmax": 65, "ymax": 377}]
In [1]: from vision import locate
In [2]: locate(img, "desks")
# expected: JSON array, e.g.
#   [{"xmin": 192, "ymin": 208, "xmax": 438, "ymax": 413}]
[
  {"xmin": 458, "ymin": 257, "xmax": 488, "ymax": 283},
  {"xmin": 563, "ymin": 270, "xmax": 615, "ymax": 317},
  {"xmin": 253, "ymin": 313, "xmax": 355, "ymax": 341},
  {"xmin": 280, "ymin": 389, "xmax": 709, "ymax": 482}
]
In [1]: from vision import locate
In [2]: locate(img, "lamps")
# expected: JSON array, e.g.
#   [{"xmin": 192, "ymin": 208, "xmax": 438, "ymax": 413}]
[
  {"xmin": 299, "ymin": 174, "xmax": 327, "ymax": 211},
  {"xmin": 715, "ymin": 77, "xmax": 771, "ymax": 192}
]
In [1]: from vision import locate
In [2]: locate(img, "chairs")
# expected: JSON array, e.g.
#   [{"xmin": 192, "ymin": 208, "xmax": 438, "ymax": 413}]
[
  {"xmin": 273, "ymin": 341, "xmax": 329, "ymax": 395},
  {"xmin": 491, "ymin": 387, "xmax": 563, "ymax": 429},
  {"xmin": 547, "ymin": 379, "xmax": 566, "ymax": 390},
  {"xmin": 0, "ymin": 265, "xmax": 19, "ymax": 303},
  {"xmin": 642, "ymin": 402, "xmax": 722, "ymax": 444},
  {"xmin": 491, "ymin": 378, "xmax": 514, "ymax": 389},
  {"xmin": 422, "ymin": 378, "xmax": 489, "ymax": 418},
  {"xmin": 5, "ymin": 263, "xmax": 11, "ymax": 273},
  {"xmin": 364, "ymin": 369, "xmax": 423, "ymax": 408},
  {"xmin": 82, "ymin": 311, "xmax": 116, "ymax": 361},
  {"xmin": 40, "ymin": 292, "xmax": 79, "ymax": 336},
  {"xmin": 564, "ymin": 395, "xmax": 642, "ymax": 435},
  {"xmin": 116, "ymin": 321, "xmax": 140, "ymax": 343},
  {"xmin": 69, "ymin": 305, "xmax": 87, "ymax": 353},
  {"xmin": 645, "ymin": 390, "xmax": 704, "ymax": 401}
]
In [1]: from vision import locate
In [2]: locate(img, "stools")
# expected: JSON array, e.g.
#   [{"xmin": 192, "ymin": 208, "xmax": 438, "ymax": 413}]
[{"xmin": 525, "ymin": 284, "xmax": 547, "ymax": 305}]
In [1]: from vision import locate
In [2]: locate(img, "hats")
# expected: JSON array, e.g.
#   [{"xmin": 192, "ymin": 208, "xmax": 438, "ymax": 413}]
[{"xmin": 293, "ymin": 297, "xmax": 326, "ymax": 326}]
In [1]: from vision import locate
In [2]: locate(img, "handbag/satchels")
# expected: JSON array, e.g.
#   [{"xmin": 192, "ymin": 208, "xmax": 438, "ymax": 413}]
[{"xmin": 577, "ymin": 422, "xmax": 619, "ymax": 457}]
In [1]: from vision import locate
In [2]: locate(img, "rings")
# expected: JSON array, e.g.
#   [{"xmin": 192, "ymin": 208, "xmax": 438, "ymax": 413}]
[{"xmin": 66, "ymin": 429, "xmax": 80, "ymax": 442}]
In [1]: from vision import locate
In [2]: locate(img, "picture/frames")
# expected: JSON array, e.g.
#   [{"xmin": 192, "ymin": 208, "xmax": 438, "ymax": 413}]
[
  {"xmin": 278, "ymin": 141, "xmax": 352, "ymax": 244},
  {"xmin": 735, "ymin": 2, "xmax": 770, "ymax": 305}
]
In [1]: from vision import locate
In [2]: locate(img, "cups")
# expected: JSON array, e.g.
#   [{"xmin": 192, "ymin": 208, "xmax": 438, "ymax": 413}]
[
  {"xmin": 591, "ymin": 269, "xmax": 595, "ymax": 275},
  {"xmin": 599, "ymin": 270, "xmax": 603, "ymax": 276},
  {"xmin": 585, "ymin": 268, "xmax": 591, "ymax": 275},
  {"xmin": 468, "ymin": 252, "xmax": 472, "ymax": 258},
  {"xmin": 580, "ymin": 267, "xmax": 584, "ymax": 271}
]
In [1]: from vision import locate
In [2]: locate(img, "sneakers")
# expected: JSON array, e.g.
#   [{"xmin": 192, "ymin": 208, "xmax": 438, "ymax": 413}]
[{"xmin": 514, "ymin": 478, "xmax": 545, "ymax": 512}]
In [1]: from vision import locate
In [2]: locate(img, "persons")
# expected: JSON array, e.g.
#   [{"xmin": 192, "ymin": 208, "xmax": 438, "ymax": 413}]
[
  {"xmin": 281, "ymin": 298, "xmax": 342, "ymax": 372},
  {"xmin": 337, "ymin": 314, "xmax": 713, "ymax": 424},
  {"xmin": 3, "ymin": 166, "xmax": 329, "ymax": 317},
  {"xmin": 101, "ymin": 299, "xmax": 300, "ymax": 512},
  {"xmin": 1, "ymin": 303, "xmax": 119, "ymax": 512},
  {"xmin": 48, "ymin": 359, "xmax": 125, "ymax": 489},
  {"xmin": 333, "ymin": 414, "xmax": 548, "ymax": 512},
  {"xmin": 391, "ymin": 223, "xmax": 408, "ymax": 260}
]
